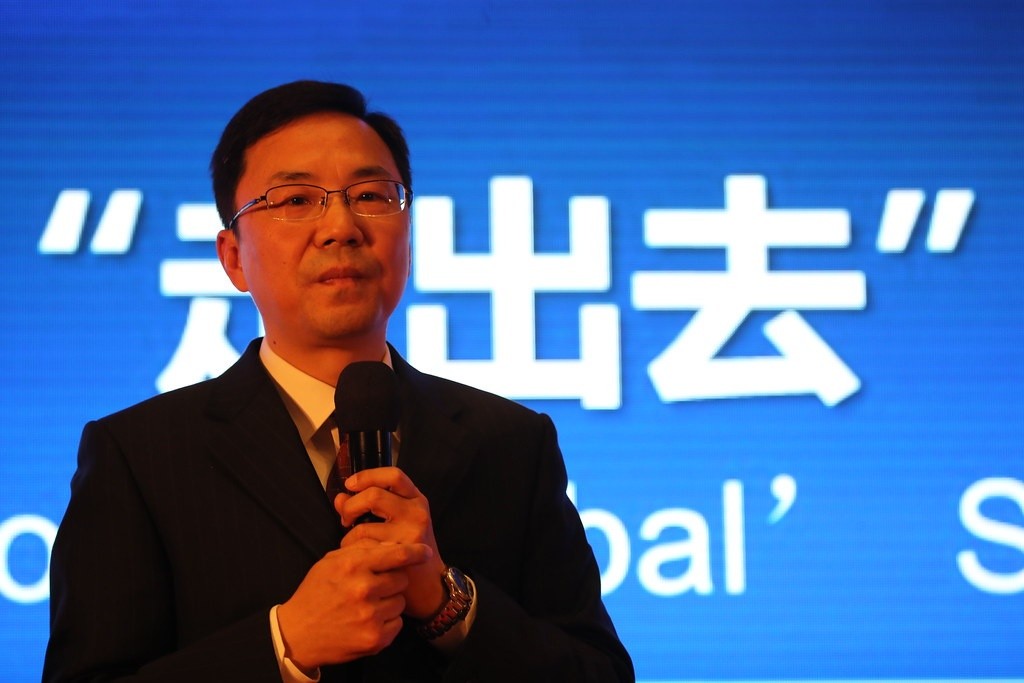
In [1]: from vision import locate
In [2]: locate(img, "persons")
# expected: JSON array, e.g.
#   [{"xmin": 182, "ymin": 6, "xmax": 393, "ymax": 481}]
[{"xmin": 39, "ymin": 80, "xmax": 637, "ymax": 683}]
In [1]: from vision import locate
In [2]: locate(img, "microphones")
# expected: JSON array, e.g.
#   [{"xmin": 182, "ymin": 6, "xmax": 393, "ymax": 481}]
[{"xmin": 334, "ymin": 361, "xmax": 400, "ymax": 527}]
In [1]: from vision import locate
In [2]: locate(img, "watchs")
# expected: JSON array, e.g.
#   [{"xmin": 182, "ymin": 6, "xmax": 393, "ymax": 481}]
[{"xmin": 412, "ymin": 565, "xmax": 473, "ymax": 642}]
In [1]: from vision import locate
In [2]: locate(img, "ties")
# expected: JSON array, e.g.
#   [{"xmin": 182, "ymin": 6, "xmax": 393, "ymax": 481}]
[{"xmin": 326, "ymin": 411, "xmax": 355, "ymax": 510}]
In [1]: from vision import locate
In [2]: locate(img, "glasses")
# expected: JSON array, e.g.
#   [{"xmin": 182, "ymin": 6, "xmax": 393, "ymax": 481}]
[{"xmin": 228, "ymin": 179, "xmax": 414, "ymax": 230}]
